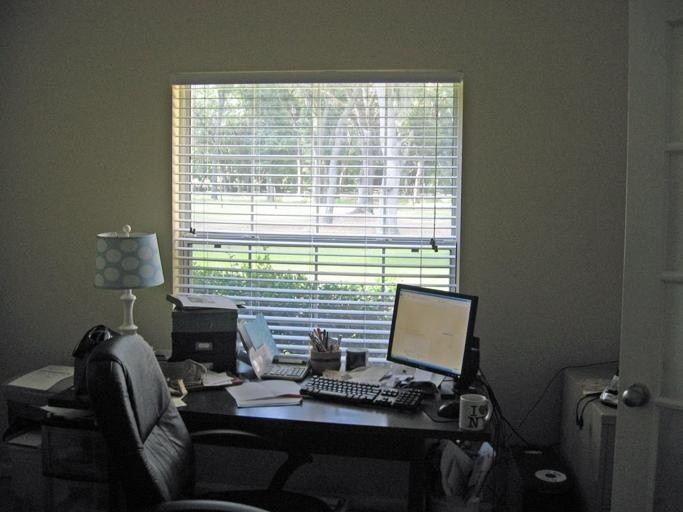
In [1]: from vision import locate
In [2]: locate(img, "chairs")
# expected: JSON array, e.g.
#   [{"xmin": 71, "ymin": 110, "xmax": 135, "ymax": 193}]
[{"xmin": 91, "ymin": 333, "xmax": 333, "ymax": 512}]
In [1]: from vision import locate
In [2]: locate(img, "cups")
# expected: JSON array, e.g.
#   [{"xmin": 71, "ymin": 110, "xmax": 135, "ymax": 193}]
[
  {"xmin": 346, "ymin": 349, "xmax": 367, "ymax": 372},
  {"xmin": 458, "ymin": 394, "xmax": 494, "ymax": 433}
]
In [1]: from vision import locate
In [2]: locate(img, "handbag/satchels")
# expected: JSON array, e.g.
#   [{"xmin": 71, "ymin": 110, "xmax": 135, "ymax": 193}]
[
  {"xmin": 73, "ymin": 324, "xmax": 122, "ymax": 396},
  {"xmin": 157, "ymin": 360, "xmax": 206, "ymax": 381}
]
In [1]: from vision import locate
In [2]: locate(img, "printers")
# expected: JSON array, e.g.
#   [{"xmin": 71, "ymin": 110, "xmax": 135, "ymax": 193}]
[{"xmin": 0, "ymin": 363, "xmax": 98, "ymax": 510}]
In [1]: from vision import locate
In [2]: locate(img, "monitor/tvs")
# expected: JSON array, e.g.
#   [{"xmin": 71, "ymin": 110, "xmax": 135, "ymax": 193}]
[{"xmin": 385, "ymin": 283, "xmax": 478, "ymax": 402}]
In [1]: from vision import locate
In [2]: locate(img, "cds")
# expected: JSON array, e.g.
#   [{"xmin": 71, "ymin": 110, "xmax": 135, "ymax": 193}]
[{"xmin": 535, "ymin": 468, "xmax": 567, "ymax": 483}]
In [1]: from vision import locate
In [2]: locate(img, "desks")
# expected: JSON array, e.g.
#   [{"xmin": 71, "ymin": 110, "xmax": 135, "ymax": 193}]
[
  {"xmin": 47, "ymin": 349, "xmax": 491, "ymax": 512},
  {"xmin": 560, "ymin": 366, "xmax": 619, "ymax": 511}
]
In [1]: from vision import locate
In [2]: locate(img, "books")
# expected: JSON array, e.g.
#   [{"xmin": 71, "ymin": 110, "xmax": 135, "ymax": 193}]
[
  {"xmin": 8, "ymin": 365, "xmax": 74, "ymax": 396},
  {"xmin": 166, "ymin": 294, "xmax": 238, "ymax": 312},
  {"xmin": 237, "ymin": 312, "xmax": 279, "ymax": 362},
  {"xmin": 225, "ymin": 379, "xmax": 303, "ymax": 407}
]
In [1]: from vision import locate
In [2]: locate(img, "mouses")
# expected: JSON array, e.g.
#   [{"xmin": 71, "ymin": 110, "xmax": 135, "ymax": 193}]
[{"xmin": 437, "ymin": 400, "xmax": 459, "ymax": 418}]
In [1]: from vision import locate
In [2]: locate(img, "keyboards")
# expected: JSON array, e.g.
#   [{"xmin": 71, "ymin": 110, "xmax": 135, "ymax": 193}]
[{"xmin": 300, "ymin": 375, "xmax": 426, "ymax": 414}]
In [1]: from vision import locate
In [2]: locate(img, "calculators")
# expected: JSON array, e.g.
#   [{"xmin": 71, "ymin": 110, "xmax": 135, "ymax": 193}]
[{"xmin": 262, "ymin": 355, "xmax": 311, "ymax": 381}]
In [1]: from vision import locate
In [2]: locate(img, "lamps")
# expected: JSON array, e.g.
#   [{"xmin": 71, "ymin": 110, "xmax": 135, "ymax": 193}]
[{"xmin": 93, "ymin": 223, "xmax": 166, "ymax": 337}]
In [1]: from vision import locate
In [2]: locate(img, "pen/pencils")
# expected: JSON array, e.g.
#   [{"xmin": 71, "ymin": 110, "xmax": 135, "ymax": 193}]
[{"xmin": 308, "ymin": 328, "xmax": 342, "ymax": 352}]
